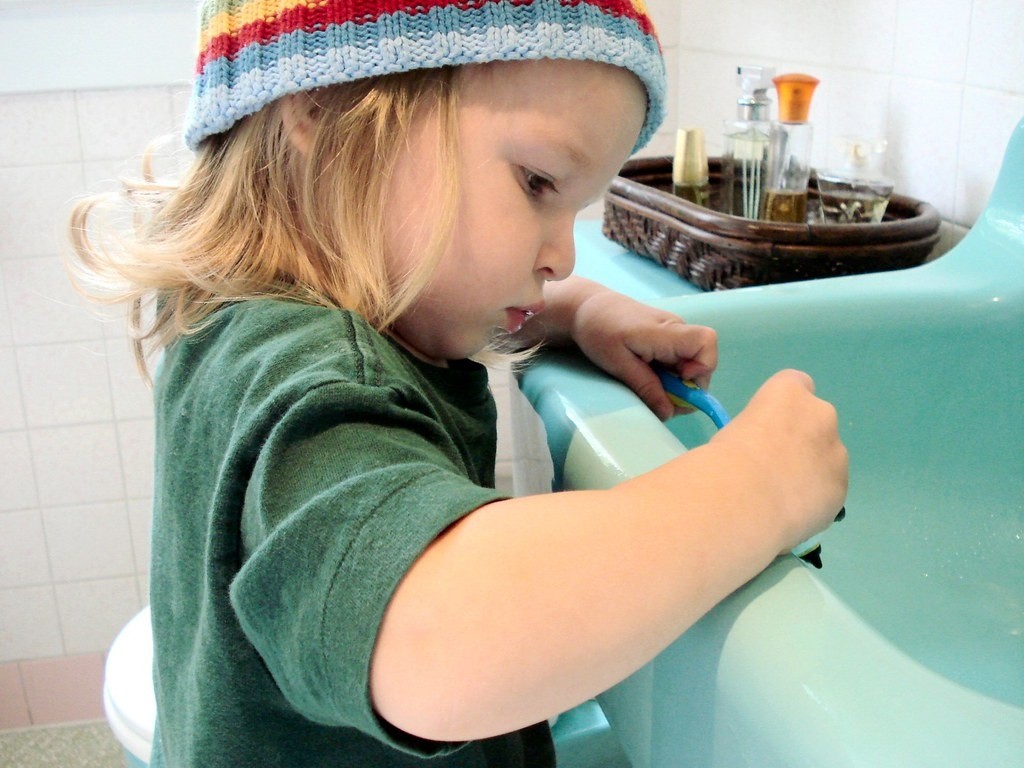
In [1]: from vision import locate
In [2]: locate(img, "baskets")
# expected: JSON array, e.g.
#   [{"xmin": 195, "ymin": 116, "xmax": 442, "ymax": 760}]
[{"xmin": 602, "ymin": 156, "xmax": 941, "ymax": 293}]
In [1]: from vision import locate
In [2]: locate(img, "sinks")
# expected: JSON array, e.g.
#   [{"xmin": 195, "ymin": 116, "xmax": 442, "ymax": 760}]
[{"xmin": 515, "ymin": 204, "xmax": 1024, "ymax": 768}]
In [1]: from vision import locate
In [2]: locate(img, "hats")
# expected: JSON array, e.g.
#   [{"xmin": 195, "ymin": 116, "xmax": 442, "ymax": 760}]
[{"xmin": 184, "ymin": 0, "xmax": 664, "ymax": 155}]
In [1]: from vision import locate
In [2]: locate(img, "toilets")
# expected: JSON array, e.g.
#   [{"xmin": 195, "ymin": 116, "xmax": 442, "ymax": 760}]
[{"xmin": 100, "ymin": 350, "xmax": 554, "ymax": 768}]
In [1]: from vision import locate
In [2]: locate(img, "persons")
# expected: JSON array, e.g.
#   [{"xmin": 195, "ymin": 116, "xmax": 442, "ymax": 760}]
[{"xmin": 61, "ymin": 0, "xmax": 847, "ymax": 767}]
